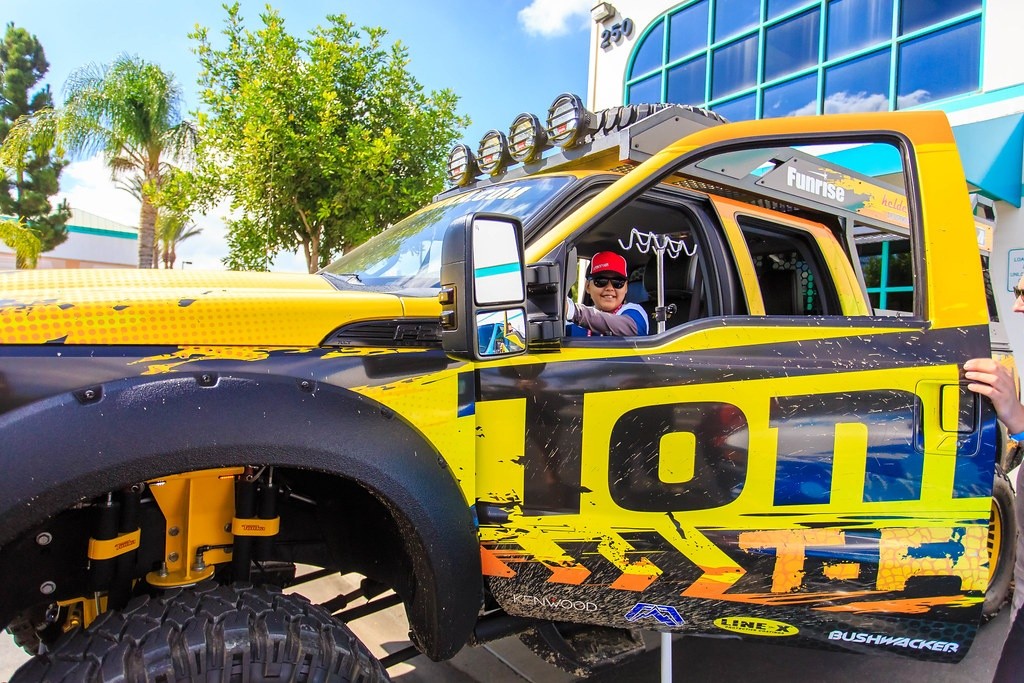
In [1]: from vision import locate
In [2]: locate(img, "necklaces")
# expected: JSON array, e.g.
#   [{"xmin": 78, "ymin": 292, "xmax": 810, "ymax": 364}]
[{"xmin": 587, "ymin": 303, "xmax": 623, "ymax": 337}]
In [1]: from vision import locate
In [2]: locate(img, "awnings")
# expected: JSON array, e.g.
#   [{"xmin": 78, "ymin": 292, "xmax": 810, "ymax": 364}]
[{"xmin": 791, "ymin": 83, "xmax": 1024, "ymax": 209}]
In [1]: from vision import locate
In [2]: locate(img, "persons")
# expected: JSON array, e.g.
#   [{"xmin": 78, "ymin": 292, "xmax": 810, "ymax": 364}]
[
  {"xmin": 502, "ymin": 322, "xmax": 514, "ymax": 349},
  {"xmin": 963, "ymin": 271, "xmax": 1024, "ymax": 446},
  {"xmin": 563, "ymin": 252, "xmax": 650, "ymax": 337}
]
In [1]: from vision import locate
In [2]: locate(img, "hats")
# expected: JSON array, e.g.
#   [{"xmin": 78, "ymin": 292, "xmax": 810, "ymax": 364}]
[{"xmin": 585, "ymin": 251, "xmax": 627, "ymax": 277}]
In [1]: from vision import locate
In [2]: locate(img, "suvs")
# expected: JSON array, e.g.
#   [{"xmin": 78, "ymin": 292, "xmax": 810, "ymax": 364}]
[{"xmin": 0, "ymin": 91, "xmax": 1023, "ymax": 683}]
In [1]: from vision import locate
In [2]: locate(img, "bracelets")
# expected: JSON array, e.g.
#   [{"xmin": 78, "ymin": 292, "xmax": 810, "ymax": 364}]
[{"xmin": 1006, "ymin": 429, "xmax": 1024, "ymax": 444}]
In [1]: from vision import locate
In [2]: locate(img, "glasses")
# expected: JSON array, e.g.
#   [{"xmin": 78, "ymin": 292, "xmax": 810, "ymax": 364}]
[
  {"xmin": 1013, "ymin": 286, "xmax": 1024, "ymax": 302},
  {"xmin": 589, "ymin": 277, "xmax": 628, "ymax": 288}
]
